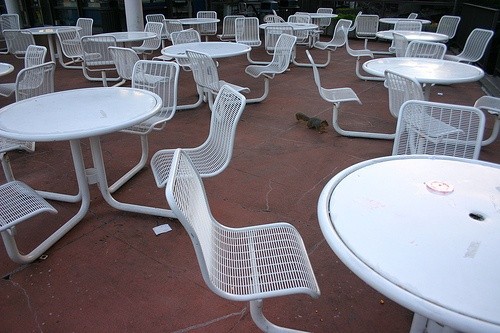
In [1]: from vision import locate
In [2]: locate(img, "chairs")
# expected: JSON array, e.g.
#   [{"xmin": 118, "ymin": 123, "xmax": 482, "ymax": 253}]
[{"xmin": 0, "ymin": 8, "xmax": 500, "ymax": 333}]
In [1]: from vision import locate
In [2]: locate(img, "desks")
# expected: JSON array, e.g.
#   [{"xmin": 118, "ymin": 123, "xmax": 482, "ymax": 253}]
[
  {"xmin": 161, "ymin": 42, "xmax": 252, "ymax": 95},
  {"xmin": 380, "ymin": 18, "xmax": 431, "ymax": 24},
  {"xmin": 259, "ymin": 22, "xmax": 319, "ymax": 57},
  {"xmin": 317, "ymin": 154, "xmax": 500, "ymax": 333},
  {"xmin": 294, "ymin": 13, "xmax": 339, "ymax": 24},
  {"xmin": 170, "ymin": 18, "xmax": 220, "ymax": 29},
  {"xmin": 376, "ymin": 31, "xmax": 449, "ymax": 42},
  {"xmin": 21, "ymin": 26, "xmax": 83, "ymax": 62},
  {"xmin": 0, "ymin": 62, "xmax": 14, "ymax": 77},
  {"xmin": 88, "ymin": 32, "xmax": 157, "ymax": 48},
  {"xmin": 362, "ymin": 57, "xmax": 485, "ymax": 101},
  {"xmin": 0, "ymin": 87, "xmax": 163, "ymax": 210}
]
